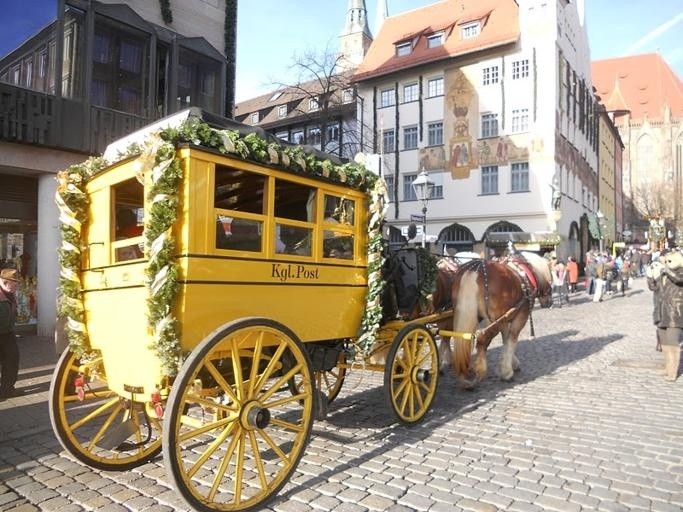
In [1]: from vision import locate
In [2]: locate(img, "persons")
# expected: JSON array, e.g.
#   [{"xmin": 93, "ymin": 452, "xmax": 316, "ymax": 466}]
[
  {"xmin": 0, "ymin": 267, "xmax": 20, "ymax": 401},
  {"xmin": 418, "ymin": 135, "xmax": 513, "ymax": 172},
  {"xmin": 647, "ymin": 250, "xmax": 683, "ymax": 382},
  {"xmin": 544, "ymin": 245, "xmax": 683, "ymax": 307},
  {"xmin": 116, "ymin": 209, "xmax": 144, "ymax": 238}
]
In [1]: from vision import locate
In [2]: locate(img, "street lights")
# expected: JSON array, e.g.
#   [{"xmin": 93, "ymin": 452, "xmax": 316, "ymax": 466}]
[{"xmin": 410, "ymin": 166, "xmax": 436, "ymax": 249}]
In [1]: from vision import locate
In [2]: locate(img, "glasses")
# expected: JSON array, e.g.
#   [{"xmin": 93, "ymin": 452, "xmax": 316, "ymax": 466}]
[{"xmin": 664, "ymin": 260, "xmax": 672, "ymax": 263}]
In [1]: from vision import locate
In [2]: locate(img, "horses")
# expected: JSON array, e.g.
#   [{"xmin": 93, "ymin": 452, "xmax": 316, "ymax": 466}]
[{"xmin": 372, "ymin": 251, "xmax": 558, "ymax": 392}]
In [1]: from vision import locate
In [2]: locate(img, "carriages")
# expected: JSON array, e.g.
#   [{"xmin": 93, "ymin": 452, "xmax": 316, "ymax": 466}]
[{"xmin": 48, "ymin": 108, "xmax": 553, "ymax": 511}]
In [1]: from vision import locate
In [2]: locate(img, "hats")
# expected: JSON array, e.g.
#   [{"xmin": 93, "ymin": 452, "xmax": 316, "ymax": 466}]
[{"xmin": 0, "ymin": 268, "xmax": 20, "ymax": 282}]
